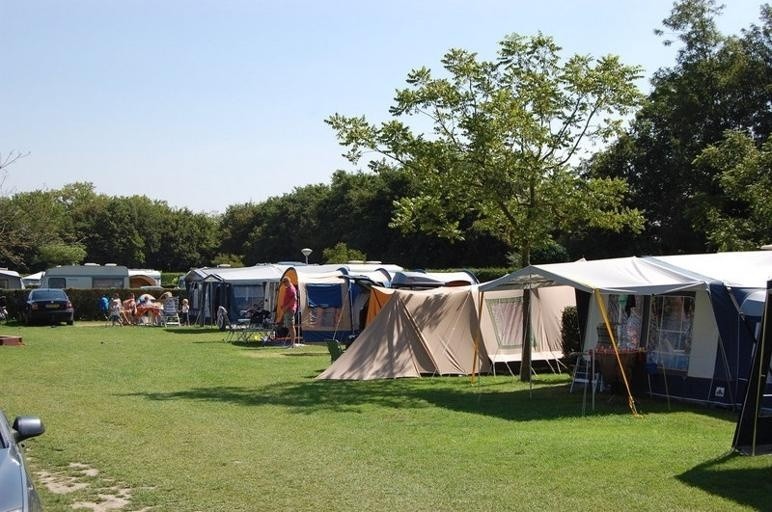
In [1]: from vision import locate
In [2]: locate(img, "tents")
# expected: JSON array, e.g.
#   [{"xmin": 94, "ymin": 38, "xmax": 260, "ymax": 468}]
[
  {"xmin": 0, "ymin": 269, "xmax": 26, "ymax": 291},
  {"xmin": 273, "ymin": 267, "xmax": 479, "ymax": 342},
  {"xmin": 20, "ymin": 270, "xmax": 46, "ymax": 286},
  {"xmin": 476, "ymin": 246, "xmax": 772, "ymax": 412},
  {"xmin": 185, "ymin": 265, "xmax": 402, "ymax": 325},
  {"xmin": 314, "ymin": 283, "xmax": 576, "ymax": 379}
]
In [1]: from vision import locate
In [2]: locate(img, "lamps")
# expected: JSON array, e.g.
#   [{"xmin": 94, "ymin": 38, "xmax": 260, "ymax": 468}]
[
  {"xmin": 325, "ymin": 339, "xmax": 345, "ymax": 364},
  {"xmin": 219, "ymin": 306, "xmax": 247, "ymax": 343},
  {"xmin": 158, "ymin": 297, "xmax": 181, "ymax": 328}
]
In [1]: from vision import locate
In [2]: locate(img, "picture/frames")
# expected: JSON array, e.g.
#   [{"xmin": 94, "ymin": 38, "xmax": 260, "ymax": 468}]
[{"xmin": 237, "ymin": 318, "xmax": 273, "ymax": 343}]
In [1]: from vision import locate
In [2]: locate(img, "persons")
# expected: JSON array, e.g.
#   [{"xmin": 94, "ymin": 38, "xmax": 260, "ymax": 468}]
[
  {"xmin": 280, "ymin": 277, "xmax": 297, "ymax": 348},
  {"xmin": 105, "ymin": 290, "xmax": 173, "ymax": 329},
  {"xmin": 181, "ymin": 299, "xmax": 190, "ymax": 326}
]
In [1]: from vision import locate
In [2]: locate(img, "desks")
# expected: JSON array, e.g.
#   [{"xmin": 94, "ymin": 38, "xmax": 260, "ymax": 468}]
[{"xmin": 301, "ymin": 247, "xmax": 314, "ymax": 264}]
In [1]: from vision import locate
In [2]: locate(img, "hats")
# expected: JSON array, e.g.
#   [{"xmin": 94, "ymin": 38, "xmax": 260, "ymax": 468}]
[
  {"xmin": 16, "ymin": 287, "xmax": 77, "ymax": 326},
  {"xmin": 0, "ymin": 400, "xmax": 48, "ymax": 511}
]
